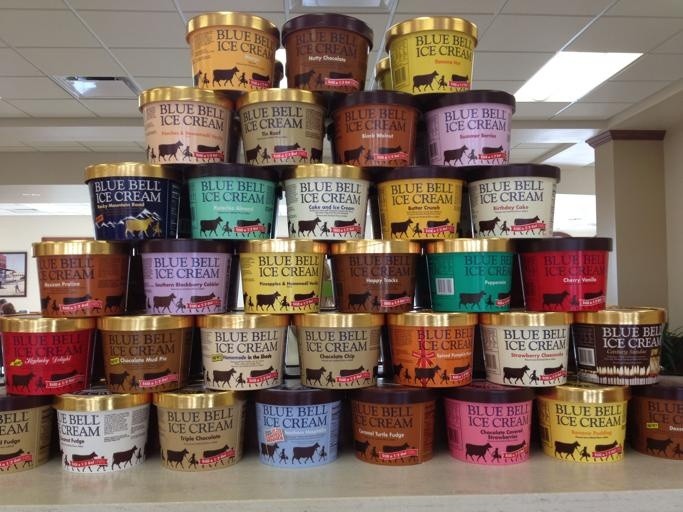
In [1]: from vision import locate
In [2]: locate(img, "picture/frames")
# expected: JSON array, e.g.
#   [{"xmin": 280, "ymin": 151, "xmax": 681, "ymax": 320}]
[{"xmin": 0, "ymin": 252, "xmax": 27, "ymax": 297}]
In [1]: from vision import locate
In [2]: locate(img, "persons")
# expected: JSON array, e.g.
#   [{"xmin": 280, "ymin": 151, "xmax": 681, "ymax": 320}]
[
  {"xmin": 1, "ymin": 303, "xmax": 17, "ymax": 315},
  {"xmin": 0, "ymin": 298, "xmax": 7, "ymax": 316},
  {"xmin": 14, "ymin": 281, "xmax": 21, "ymax": 294}
]
